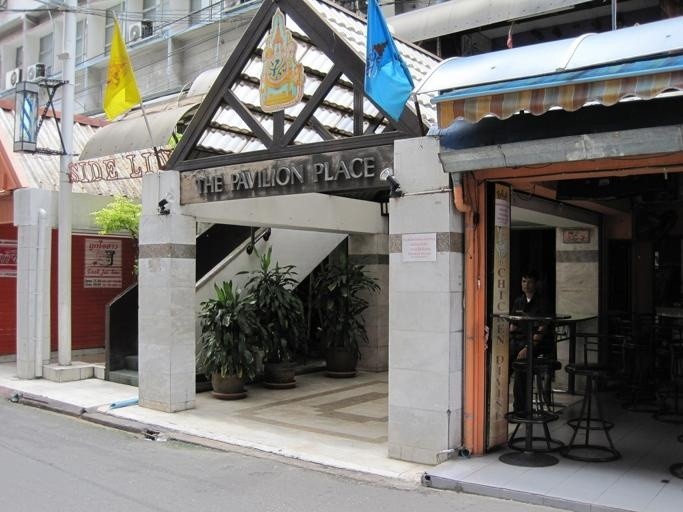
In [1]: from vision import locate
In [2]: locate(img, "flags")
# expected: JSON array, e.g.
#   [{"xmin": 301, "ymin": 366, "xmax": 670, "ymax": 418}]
[
  {"xmin": 103, "ymin": 15, "xmax": 142, "ymax": 121},
  {"xmin": 363, "ymin": 0, "xmax": 414, "ymax": 125}
]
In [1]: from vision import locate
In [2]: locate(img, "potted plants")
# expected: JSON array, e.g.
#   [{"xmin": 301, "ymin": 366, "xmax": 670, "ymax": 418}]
[{"xmin": 195, "ymin": 245, "xmax": 382, "ymax": 400}]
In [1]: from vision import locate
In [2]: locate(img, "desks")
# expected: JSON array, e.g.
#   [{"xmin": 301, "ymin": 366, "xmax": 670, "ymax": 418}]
[{"xmin": 494, "ymin": 312, "xmax": 600, "ymax": 468}]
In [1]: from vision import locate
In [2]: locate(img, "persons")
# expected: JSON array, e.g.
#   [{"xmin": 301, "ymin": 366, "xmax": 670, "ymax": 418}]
[{"xmin": 509, "ymin": 269, "xmax": 553, "ymax": 417}]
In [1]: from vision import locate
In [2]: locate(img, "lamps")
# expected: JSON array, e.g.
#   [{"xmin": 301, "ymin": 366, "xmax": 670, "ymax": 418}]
[
  {"xmin": 379, "ymin": 167, "xmax": 404, "ymax": 197},
  {"xmin": 156, "ymin": 191, "xmax": 176, "ymax": 216}
]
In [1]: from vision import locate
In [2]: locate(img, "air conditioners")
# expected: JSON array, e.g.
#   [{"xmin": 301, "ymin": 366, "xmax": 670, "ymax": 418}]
[
  {"xmin": 5, "ymin": 68, "xmax": 22, "ymax": 90},
  {"xmin": 127, "ymin": 19, "xmax": 154, "ymax": 47},
  {"xmin": 26, "ymin": 63, "xmax": 46, "ymax": 82}
]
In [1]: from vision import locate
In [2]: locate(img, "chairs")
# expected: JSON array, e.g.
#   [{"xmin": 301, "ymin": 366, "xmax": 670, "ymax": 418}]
[{"xmin": 563, "ymin": 310, "xmax": 683, "ymax": 479}]
